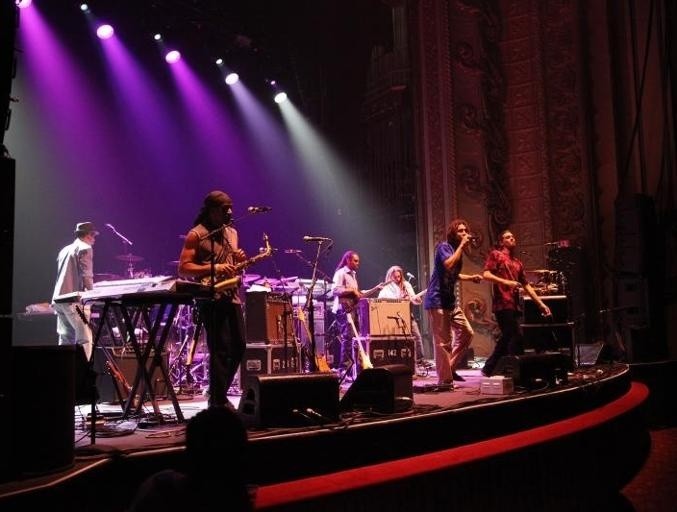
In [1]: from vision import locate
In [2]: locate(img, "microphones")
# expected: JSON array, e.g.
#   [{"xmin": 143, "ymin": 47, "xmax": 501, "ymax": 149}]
[
  {"xmin": 407, "ymin": 272, "xmax": 415, "ymax": 278},
  {"xmin": 259, "ymin": 247, "xmax": 277, "ymax": 253},
  {"xmin": 291, "ymin": 408, "xmax": 312, "ymax": 422},
  {"xmin": 306, "ymin": 407, "xmax": 327, "ymax": 421},
  {"xmin": 246, "ymin": 205, "xmax": 273, "ymax": 213},
  {"xmin": 284, "ymin": 249, "xmax": 303, "ymax": 253},
  {"xmin": 387, "ymin": 316, "xmax": 399, "ymax": 320},
  {"xmin": 468, "ymin": 236, "xmax": 480, "ymax": 240},
  {"xmin": 304, "ymin": 235, "xmax": 325, "ymax": 242},
  {"xmin": 541, "ymin": 313, "xmax": 551, "ymax": 321},
  {"xmin": 104, "ymin": 223, "xmax": 133, "ymax": 247}
]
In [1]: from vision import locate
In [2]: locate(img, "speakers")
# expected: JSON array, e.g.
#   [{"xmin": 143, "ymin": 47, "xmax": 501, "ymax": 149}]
[
  {"xmin": 341, "ymin": 363, "xmax": 415, "ymax": 415},
  {"xmin": 617, "ymin": 277, "xmax": 657, "ymax": 329},
  {"xmin": 614, "ymin": 192, "xmax": 654, "ymax": 274},
  {"xmin": 239, "ymin": 371, "xmax": 340, "ymax": 432},
  {"xmin": 0, "ymin": 310, "xmax": 76, "ymax": 482},
  {"xmin": 504, "ymin": 355, "xmax": 569, "ymax": 389},
  {"xmin": 623, "ymin": 328, "xmax": 668, "ymax": 378}
]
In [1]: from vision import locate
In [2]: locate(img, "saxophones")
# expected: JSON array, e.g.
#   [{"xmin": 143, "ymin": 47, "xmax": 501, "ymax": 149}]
[{"xmin": 201, "ymin": 231, "xmax": 272, "ymax": 292}]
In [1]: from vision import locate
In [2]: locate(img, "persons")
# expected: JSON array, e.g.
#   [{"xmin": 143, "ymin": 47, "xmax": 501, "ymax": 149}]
[
  {"xmin": 126, "ymin": 408, "xmax": 253, "ymax": 508},
  {"xmin": 378, "ymin": 265, "xmax": 432, "ymax": 367},
  {"xmin": 50, "ymin": 221, "xmax": 99, "ymax": 362},
  {"xmin": 330, "ymin": 251, "xmax": 384, "ymax": 382},
  {"xmin": 178, "ymin": 191, "xmax": 247, "ymax": 417},
  {"xmin": 423, "ymin": 220, "xmax": 483, "ymax": 390},
  {"xmin": 482, "ymin": 230, "xmax": 551, "ymax": 377}
]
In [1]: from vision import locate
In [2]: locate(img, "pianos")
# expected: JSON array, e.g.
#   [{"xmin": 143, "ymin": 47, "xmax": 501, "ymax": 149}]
[{"xmin": 52, "ymin": 275, "xmax": 192, "ymax": 303}]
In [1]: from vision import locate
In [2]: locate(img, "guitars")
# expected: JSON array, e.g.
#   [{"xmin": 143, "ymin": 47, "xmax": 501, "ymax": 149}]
[{"xmin": 340, "ymin": 283, "xmax": 384, "ymax": 314}]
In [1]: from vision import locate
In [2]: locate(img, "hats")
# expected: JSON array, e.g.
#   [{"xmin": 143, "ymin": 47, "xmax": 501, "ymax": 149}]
[{"xmin": 74, "ymin": 222, "xmax": 100, "ymax": 235}]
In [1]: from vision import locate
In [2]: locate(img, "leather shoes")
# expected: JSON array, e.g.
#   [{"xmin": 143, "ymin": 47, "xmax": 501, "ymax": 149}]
[{"xmin": 452, "ymin": 373, "xmax": 466, "ymax": 382}]
